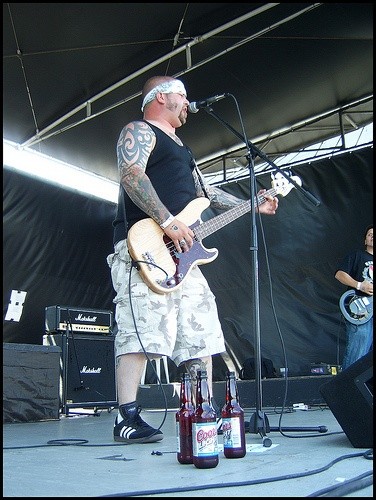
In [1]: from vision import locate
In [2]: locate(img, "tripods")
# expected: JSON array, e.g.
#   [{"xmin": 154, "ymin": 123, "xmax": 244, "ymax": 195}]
[{"xmin": 205, "ymin": 106, "xmax": 328, "ymax": 447}]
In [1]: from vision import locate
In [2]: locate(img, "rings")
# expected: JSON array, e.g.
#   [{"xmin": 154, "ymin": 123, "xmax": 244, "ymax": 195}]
[{"xmin": 180, "ymin": 240, "xmax": 186, "ymax": 247}]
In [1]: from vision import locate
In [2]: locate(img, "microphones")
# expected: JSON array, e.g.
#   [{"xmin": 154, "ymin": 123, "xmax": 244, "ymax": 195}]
[{"xmin": 188, "ymin": 93, "xmax": 229, "ymax": 113}]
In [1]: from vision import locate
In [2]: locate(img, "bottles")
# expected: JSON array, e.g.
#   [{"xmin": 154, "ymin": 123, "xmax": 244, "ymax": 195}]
[
  {"xmin": 191, "ymin": 370, "xmax": 219, "ymax": 466},
  {"xmin": 176, "ymin": 372, "xmax": 196, "ymax": 463},
  {"xmin": 221, "ymin": 371, "xmax": 245, "ymax": 457}
]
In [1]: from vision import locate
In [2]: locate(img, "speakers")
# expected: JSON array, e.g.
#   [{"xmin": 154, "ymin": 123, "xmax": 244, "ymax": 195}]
[
  {"xmin": 42, "ymin": 334, "xmax": 120, "ymax": 408},
  {"xmin": 320, "ymin": 349, "xmax": 373, "ymax": 449}
]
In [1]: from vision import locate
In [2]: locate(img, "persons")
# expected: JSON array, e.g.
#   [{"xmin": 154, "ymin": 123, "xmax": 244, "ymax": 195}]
[
  {"xmin": 334, "ymin": 225, "xmax": 373, "ymax": 371},
  {"xmin": 107, "ymin": 77, "xmax": 278, "ymax": 444}
]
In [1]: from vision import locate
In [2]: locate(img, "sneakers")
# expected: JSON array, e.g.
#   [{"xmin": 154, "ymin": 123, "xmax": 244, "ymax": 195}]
[
  {"xmin": 113, "ymin": 401, "xmax": 163, "ymax": 442},
  {"xmin": 208, "ymin": 397, "xmax": 251, "ymax": 434}
]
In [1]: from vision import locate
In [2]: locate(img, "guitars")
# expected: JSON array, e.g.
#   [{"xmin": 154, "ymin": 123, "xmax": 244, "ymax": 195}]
[
  {"xmin": 338, "ymin": 281, "xmax": 374, "ymax": 326},
  {"xmin": 125, "ymin": 173, "xmax": 303, "ymax": 296}
]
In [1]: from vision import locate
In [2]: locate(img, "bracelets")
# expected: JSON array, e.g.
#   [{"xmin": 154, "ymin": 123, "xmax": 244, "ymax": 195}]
[
  {"xmin": 160, "ymin": 215, "xmax": 174, "ymax": 229},
  {"xmin": 356, "ymin": 282, "xmax": 361, "ymax": 290}
]
